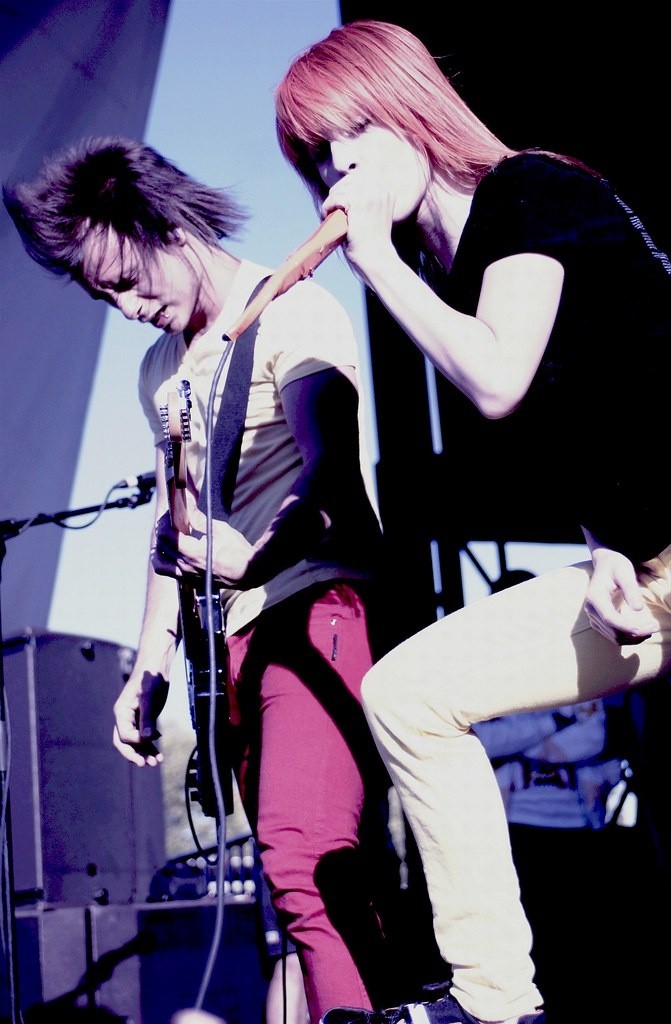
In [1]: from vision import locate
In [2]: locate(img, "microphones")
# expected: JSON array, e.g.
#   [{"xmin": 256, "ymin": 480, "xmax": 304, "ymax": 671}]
[
  {"xmin": 224, "ymin": 209, "xmax": 349, "ymax": 341},
  {"xmin": 116, "ymin": 471, "xmax": 158, "ymax": 489}
]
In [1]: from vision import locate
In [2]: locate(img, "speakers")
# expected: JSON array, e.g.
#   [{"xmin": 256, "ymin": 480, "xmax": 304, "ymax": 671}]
[{"xmin": 0, "ymin": 624, "xmax": 170, "ymax": 911}]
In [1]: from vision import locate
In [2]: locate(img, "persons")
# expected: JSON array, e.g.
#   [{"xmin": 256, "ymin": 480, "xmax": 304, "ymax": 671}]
[
  {"xmin": 275, "ymin": 20, "xmax": 670, "ymax": 1024},
  {"xmin": 0, "ymin": 137, "xmax": 383, "ymax": 1024}
]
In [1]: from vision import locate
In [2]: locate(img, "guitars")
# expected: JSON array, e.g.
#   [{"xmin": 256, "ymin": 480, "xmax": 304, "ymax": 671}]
[{"xmin": 156, "ymin": 376, "xmax": 239, "ymax": 819}]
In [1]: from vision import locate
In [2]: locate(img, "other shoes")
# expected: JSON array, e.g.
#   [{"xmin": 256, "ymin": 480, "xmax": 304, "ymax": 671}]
[{"xmin": 392, "ymin": 992, "xmax": 547, "ymax": 1024}]
